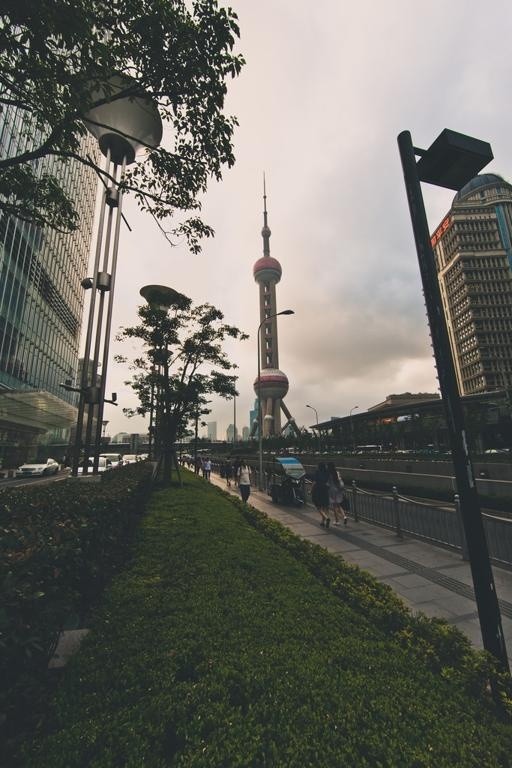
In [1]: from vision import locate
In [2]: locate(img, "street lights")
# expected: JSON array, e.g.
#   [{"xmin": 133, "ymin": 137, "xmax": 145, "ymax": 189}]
[
  {"xmin": 306, "ymin": 404, "xmax": 318, "ymax": 425},
  {"xmin": 102, "ymin": 421, "xmax": 109, "ymax": 437},
  {"xmin": 194, "ymin": 410, "xmax": 209, "ymax": 474},
  {"xmin": 397, "ymin": 128, "xmax": 512, "ymax": 699},
  {"xmin": 350, "ymin": 406, "xmax": 359, "ymax": 432},
  {"xmin": 59, "ymin": 69, "xmax": 162, "ymax": 484},
  {"xmin": 257, "ymin": 309, "xmax": 295, "ymax": 491}
]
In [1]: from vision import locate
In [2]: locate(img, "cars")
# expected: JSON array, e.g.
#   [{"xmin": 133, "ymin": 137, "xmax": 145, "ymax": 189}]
[
  {"xmin": 99, "ymin": 453, "xmax": 123, "ymax": 468},
  {"xmin": 70, "ymin": 456, "xmax": 112, "ymax": 474},
  {"xmin": 15, "ymin": 456, "xmax": 59, "ymax": 477},
  {"xmin": 122, "ymin": 453, "xmax": 149, "ymax": 466}
]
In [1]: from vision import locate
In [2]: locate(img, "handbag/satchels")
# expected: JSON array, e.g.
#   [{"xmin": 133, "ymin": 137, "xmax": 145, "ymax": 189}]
[{"xmin": 339, "ymin": 495, "xmax": 349, "ymax": 512}]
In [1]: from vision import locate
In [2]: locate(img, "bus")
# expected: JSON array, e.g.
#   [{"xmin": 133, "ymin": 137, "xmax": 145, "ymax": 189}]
[{"xmin": 352, "ymin": 444, "xmax": 392, "ymax": 453}]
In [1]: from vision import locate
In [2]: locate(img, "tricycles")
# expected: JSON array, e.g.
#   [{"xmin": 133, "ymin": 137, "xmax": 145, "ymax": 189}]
[{"xmin": 267, "ymin": 457, "xmax": 306, "ymax": 505}]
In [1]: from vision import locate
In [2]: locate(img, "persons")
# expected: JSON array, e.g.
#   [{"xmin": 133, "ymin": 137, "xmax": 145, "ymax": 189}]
[{"xmin": 181, "ymin": 454, "xmax": 350, "ymax": 529}]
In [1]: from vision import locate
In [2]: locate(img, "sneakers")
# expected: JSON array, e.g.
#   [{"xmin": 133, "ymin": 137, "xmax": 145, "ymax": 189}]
[
  {"xmin": 325, "ymin": 518, "xmax": 330, "ymax": 529},
  {"xmin": 320, "ymin": 520, "xmax": 324, "ymax": 527},
  {"xmin": 333, "ymin": 521, "xmax": 341, "ymax": 526},
  {"xmin": 343, "ymin": 516, "xmax": 347, "ymax": 525}
]
